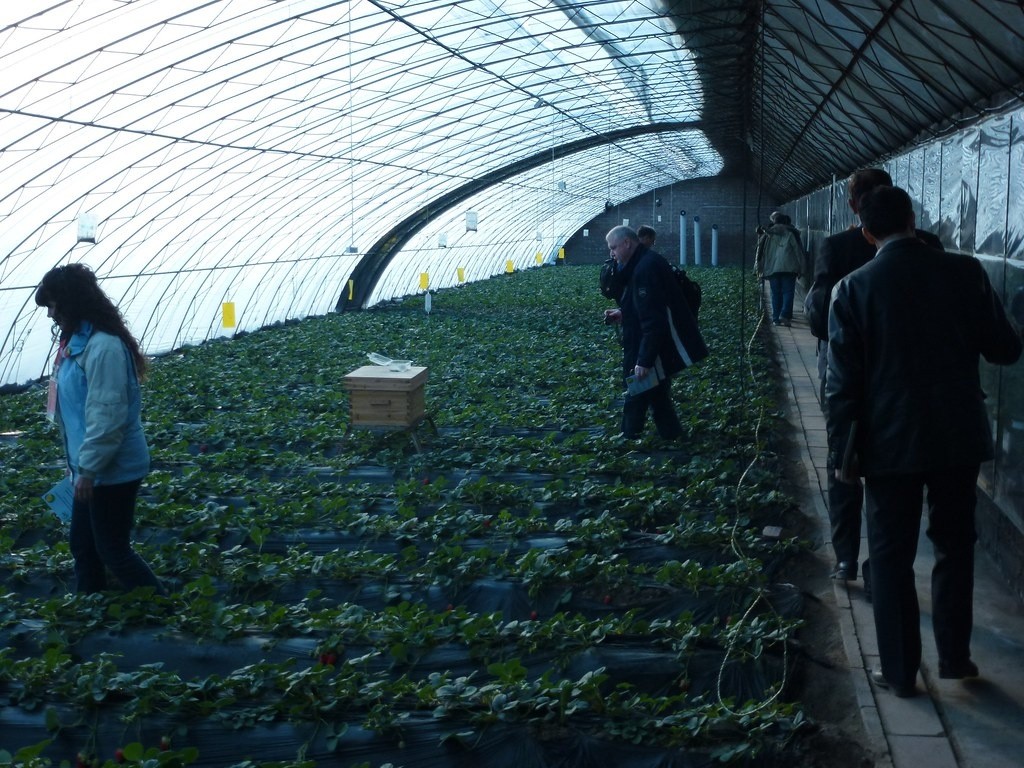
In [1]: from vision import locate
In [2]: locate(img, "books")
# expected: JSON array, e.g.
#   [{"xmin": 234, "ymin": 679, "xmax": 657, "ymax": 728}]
[
  {"xmin": 42, "ymin": 475, "xmax": 75, "ymax": 522},
  {"xmin": 625, "ymin": 367, "xmax": 658, "ymax": 395}
]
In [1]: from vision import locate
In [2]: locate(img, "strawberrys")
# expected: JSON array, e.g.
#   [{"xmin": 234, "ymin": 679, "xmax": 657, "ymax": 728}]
[
  {"xmin": 447, "ymin": 604, "xmax": 454, "ymax": 610},
  {"xmin": 319, "ymin": 653, "xmax": 337, "ymax": 665},
  {"xmin": 713, "ymin": 616, "xmax": 732, "ymax": 624},
  {"xmin": 530, "ymin": 610, "xmax": 537, "ymax": 620},
  {"xmin": 482, "ymin": 519, "xmax": 490, "ymax": 527},
  {"xmin": 604, "ymin": 595, "xmax": 613, "ymax": 605},
  {"xmin": 75, "ymin": 736, "xmax": 172, "ymax": 768},
  {"xmin": 680, "ymin": 679, "xmax": 690, "ymax": 691},
  {"xmin": 656, "ymin": 529, "xmax": 663, "ymax": 534},
  {"xmin": 423, "ymin": 479, "xmax": 428, "ymax": 485},
  {"xmin": 200, "ymin": 445, "xmax": 208, "ymax": 452}
]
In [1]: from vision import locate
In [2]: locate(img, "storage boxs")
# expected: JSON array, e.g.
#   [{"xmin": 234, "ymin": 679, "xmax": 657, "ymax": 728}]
[
  {"xmin": 368, "ymin": 351, "xmax": 415, "ymax": 371},
  {"xmin": 342, "ymin": 365, "xmax": 430, "ymax": 427}
]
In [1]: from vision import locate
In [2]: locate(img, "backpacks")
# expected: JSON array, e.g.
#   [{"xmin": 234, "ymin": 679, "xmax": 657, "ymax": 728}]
[{"xmin": 599, "ymin": 259, "xmax": 618, "ymax": 300}]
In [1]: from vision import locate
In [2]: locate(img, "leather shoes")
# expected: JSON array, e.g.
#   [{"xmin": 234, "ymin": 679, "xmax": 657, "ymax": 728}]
[
  {"xmin": 830, "ymin": 560, "xmax": 858, "ymax": 580},
  {"xmin": 872, "ymin": 662, "xmax": 915, "ymax": 697},
  {"xmin": 865, "ymin": 579, "xmax": 872, "ymax": 598},
  {"xmin": 939, "ymin": 660, "xmax": 978, "ymax": 679}
]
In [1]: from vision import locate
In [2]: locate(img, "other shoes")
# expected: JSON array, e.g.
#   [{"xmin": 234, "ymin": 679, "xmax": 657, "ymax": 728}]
[
  {"xmin": 779, "ymin": 316, "xmax": 791, "ymax": 326},
  {"xmin": 773, "ymin": 321, "xmax": 779, "ymax": 326}
]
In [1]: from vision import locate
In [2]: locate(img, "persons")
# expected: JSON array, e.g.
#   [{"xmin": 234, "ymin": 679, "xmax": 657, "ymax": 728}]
[
  {"xmin": 752, "ymin": 209, "xmax": 806, "ymax": 327},
  {"xmin": 824, "ymin": 186, "xmax": 1024, "ymax": 697},
  {"xmin": 804, "ymin": 169, "xmax": 946, "ymax": 603},
  {"xmin": 36, "ymin": 264, "xmax": 169, "ymax": 602},
  {"xmin": 616, "ymin": 226, "xmax": 656, "ymax": 309},
  {"xmin": 605, "ymin": 223, "xmax": 684, "ymax": 440}
]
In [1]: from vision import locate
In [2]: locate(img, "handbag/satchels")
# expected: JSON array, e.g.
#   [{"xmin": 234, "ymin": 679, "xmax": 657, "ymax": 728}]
[{"xmin": 670, "ymin": 264, "xmax": 701, "ymax": 324}]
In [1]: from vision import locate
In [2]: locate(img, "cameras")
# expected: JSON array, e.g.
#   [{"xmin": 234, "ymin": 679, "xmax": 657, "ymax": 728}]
[{"xmin": 604, "ymin": 316, "xmax": 616, "ymax": 326}]
